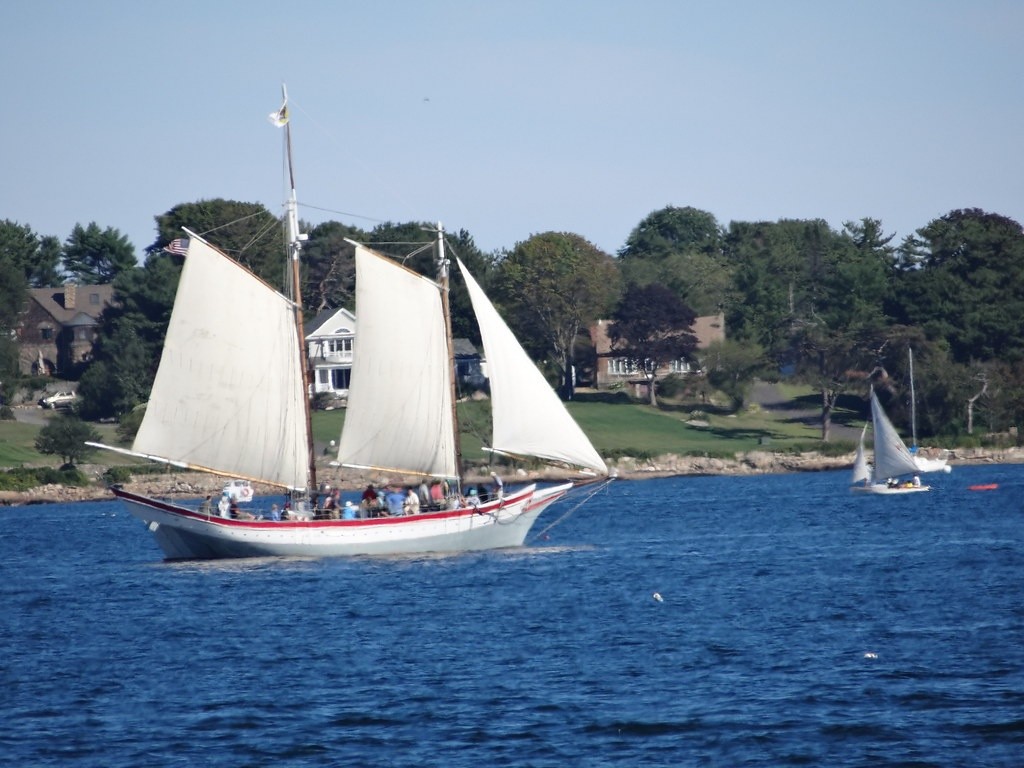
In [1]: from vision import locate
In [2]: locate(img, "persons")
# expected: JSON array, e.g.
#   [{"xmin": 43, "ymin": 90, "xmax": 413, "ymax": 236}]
[
  {"xmin": 199, "ymin": 473, "xmax": 503, "ymax": 521},
  {"xmin": 885, "ymin": 472, "xmax": 921, "ymax": 489}
]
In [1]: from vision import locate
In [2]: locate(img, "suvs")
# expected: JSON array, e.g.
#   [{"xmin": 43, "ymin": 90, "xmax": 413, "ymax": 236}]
[{"xmin": 38, "ymin": 390, "xmax": 79, "ymax": 410}]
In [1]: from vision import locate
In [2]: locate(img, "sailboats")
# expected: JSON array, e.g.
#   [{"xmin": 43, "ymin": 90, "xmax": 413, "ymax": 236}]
[
  {"xmin": 847, "ymin": 385, "xmax": 931, "ymax": 494},
  {"xmin": 83, "ymin": 79, "xmax": 621, "ymax": 558}
]
